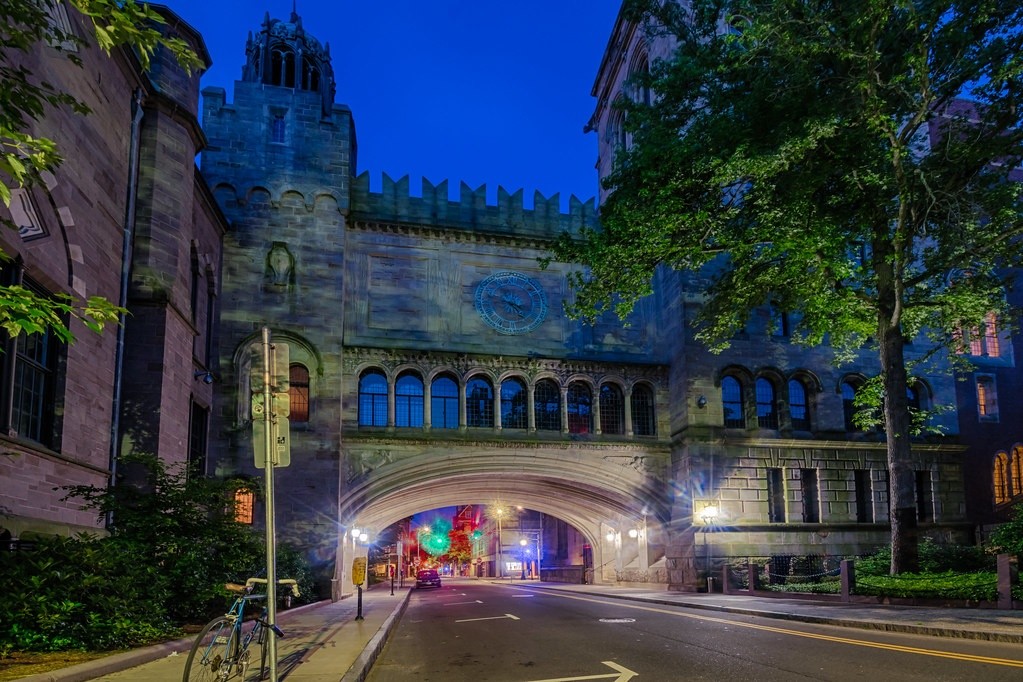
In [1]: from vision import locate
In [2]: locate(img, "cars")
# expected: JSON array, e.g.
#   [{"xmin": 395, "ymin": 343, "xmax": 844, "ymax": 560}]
[{"xmin": 414, "ymin": 570, "xmax": 442, "ymax": 589}]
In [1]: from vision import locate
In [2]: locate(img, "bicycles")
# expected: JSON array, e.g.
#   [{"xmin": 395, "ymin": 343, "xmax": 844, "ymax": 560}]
[{"xmin": 182, "ymin": 579, "xmax": 301, "ymax": 682}]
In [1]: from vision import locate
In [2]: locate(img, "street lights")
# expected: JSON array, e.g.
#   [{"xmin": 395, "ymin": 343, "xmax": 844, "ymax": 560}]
[
  {"xmin": 497, "ymin": 508, "xmax": 504, "ymax": 579},
  {"xmin": 417, "ymin": 527, "xmax": 429, "ymax": 557},
  {"xmin": 518, "ymin": 506, "xmax": 526, "ymax": 581}
]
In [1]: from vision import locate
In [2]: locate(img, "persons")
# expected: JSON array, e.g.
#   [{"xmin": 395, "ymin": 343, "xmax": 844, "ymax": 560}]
[{"xmin": 450, "ymin": 569, "xmax": 454, "ymax": 577}]
